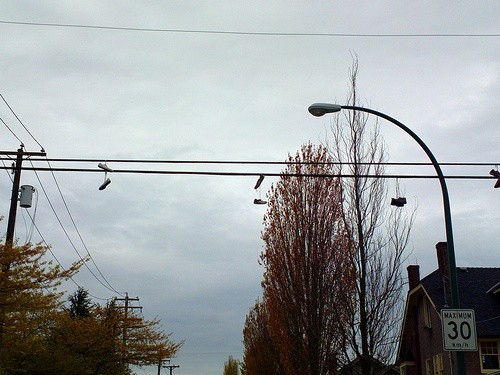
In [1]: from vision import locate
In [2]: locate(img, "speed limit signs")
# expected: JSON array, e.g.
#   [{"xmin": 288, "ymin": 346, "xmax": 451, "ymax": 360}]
[{"xmin": 442, "ymin": 308, "xmax": 479, "ymax": 351}]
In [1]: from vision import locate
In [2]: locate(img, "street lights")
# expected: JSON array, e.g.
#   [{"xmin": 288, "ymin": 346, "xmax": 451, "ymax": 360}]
[{"xmin": 310, "ymin": 103, "xmax": 467, "ymax": 375}]
